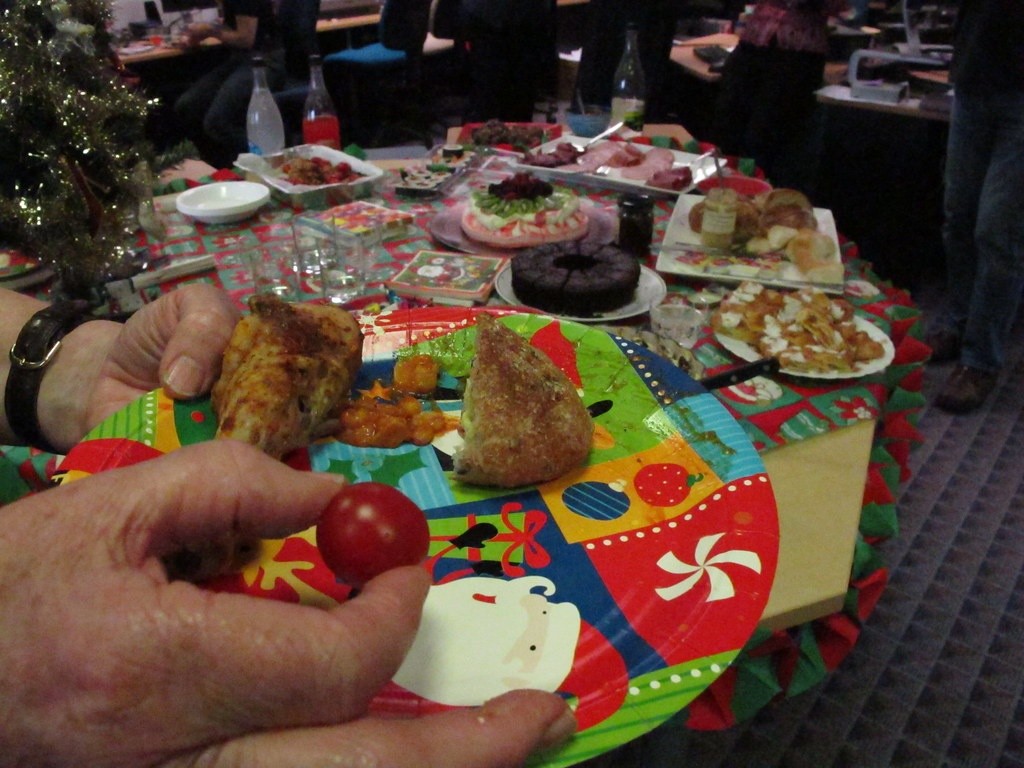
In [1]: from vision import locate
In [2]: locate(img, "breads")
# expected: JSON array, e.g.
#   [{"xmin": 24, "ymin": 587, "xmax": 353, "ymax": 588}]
[
  {"xmin": 689, "ymin": 187, "xmax": 816, "ymax": 239},
  {"xmin": 451, "ymin": 311, "xmax": 595, "ymax": 488}
]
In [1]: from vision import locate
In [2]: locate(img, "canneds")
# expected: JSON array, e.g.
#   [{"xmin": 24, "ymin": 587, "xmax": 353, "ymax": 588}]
[
  {"xmin": 613, "ymin": 192, "xmax": 654, "ymax": 249},
  {"xmin": 700, "ymin": 188, "xmax": 739, "ymax": 249}
]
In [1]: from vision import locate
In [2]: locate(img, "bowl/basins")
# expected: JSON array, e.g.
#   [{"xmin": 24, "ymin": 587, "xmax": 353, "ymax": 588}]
[
  {"xmin": 232, "ymin": 143, "xmax": 387, "ymax": 193},
  {"xmin": 146, "ymin": 25, "xmax": 192, "ymax": 49},
  {"xmin": 175, "ymin": 181, "xmax": 267, "ymax": 224}
]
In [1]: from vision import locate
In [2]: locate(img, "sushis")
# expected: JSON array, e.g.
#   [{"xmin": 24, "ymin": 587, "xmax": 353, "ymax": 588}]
[{"xmin": 395, "ymin": 144, "xmax": 476, "ymax": 189}]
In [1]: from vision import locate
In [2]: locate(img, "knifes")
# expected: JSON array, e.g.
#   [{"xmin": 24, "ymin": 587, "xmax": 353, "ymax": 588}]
[{"xmin": 648, "ymin": 240, "xmax": 789, "ymax": 262}]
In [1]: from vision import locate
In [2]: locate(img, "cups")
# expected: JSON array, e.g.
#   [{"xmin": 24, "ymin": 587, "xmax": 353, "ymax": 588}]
[
  {"xmin": 652, "ymin": 290, "xmax": 709, "ymax": 361},
  {"xmin": 248, "ymin": 210, "xmax": 382, "ymax": 304}
]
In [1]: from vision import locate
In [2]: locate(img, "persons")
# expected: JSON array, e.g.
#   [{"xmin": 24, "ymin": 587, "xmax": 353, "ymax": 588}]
[
  {"xmin": 919, "ymin": 0, "xmax": 1024, "ymax": 411},
  {"xmin": 0, "ymin": 282, "xmax": 577, "ymax": 768},
  {"xmin": 171, "ymin": 0, "xmax": 287, "ymax": 154}
]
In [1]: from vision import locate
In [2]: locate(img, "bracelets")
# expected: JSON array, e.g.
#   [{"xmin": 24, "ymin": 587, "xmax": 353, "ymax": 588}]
[{"xmin": 5, "ymin": 302, "xmax": 95, "ymax": 455}]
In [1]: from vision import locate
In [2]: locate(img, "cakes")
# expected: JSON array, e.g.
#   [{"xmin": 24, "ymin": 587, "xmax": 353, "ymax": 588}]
[
  {"xmin": 460, "ymin": 174, "xmax": 590, "ymax": 249},
  {"xmin": 510, "ymin": 241, "xmax": 641, "ymax": 316}
]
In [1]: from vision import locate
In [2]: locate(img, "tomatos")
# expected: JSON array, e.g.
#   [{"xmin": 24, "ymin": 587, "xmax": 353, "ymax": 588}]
[
  {"xmin": 311, "ymin": 157, "xmax": 349, "ymax": 184},
  {"xmin": 316, "ymin": 481, "xmax": 428, "ymax": 589}
]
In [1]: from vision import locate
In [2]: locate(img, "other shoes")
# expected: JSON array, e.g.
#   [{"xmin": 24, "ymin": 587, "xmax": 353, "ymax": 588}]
[
  {"xmin": 926, "ymin": 316, "xmax": 968, "ymax": 361},
  {"xmin": 941, "ymin": 364, "xmax": 999, "ymax": 412}
]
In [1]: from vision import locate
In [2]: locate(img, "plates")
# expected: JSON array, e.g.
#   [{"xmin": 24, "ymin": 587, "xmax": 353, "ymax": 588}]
[
  {"xmin": 506, "ymin": 132, "xmax": 730, "ymax": 194},
  {"xmin": 494, "ymin": 262, "xmax": 667, "ymax": 323},
  {"xmin": 716, "ymin": 299, "xmax": 895, "ymax": 379},
  {"xmin": 47, "ymin": 306, "xmax": 781, "ymax": 768},
  {"xmin": 390, "ymin": 143, "xmax": 522, "ymax": 191},
  {"xmin": 429, "ymin": 196, "xmax": 621, "ymax": 258},
  {"xmin": 651, "ymin": 192, "xmax": 846, "ymax": 298}
]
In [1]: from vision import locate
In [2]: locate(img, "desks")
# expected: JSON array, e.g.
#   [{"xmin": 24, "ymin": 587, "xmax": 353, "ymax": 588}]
[
  {"xmin": 0, "ymin": 116, "xmax": 916, "ymax": 640},
  {"xmin": 670, "ymin": 28, "xmax": 743, "ymax": 81},
  {"xmin": 810, "ymin": 44, "xmax": 954, "ymax": 253},
  {"xmin": 115, "ymin": 10, "xmax": 385, "ymax": 64}
]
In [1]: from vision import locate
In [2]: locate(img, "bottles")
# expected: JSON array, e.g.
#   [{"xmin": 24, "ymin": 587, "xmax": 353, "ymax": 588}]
[
  {"xmin": 301, "ymin": 53, "xmax": 341, "ymax": 152},
  {"xmin": 246, "ymin": 55, "xmax": 284, "ymax": 159},
  {"xmin": 607, "ymin": 21, "xmax": 646, "ymax": 131}
]
